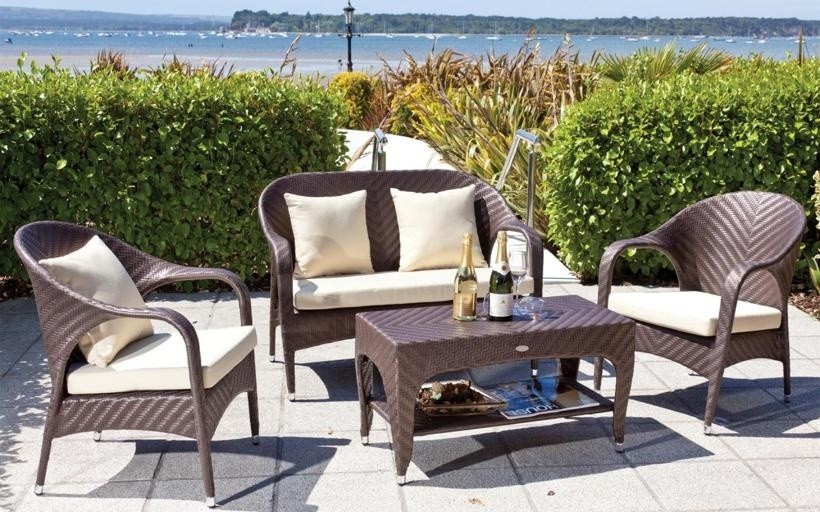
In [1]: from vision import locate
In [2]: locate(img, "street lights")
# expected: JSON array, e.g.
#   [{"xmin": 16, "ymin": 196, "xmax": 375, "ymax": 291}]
[{"xmin": 337, "ymin": 0, "xmax": 361, "ymax": 72}]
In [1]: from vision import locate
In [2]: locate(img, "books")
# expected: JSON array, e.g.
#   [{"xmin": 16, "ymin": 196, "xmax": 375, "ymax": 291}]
[{"xmin": 486, "ymin": 372, "xmax": 601, "ymax": 421}]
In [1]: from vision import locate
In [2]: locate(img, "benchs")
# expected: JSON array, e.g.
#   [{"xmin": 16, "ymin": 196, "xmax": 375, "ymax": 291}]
[{"xmin": 257, "ymin": 170, "xmax": 545, "ymax": 401}]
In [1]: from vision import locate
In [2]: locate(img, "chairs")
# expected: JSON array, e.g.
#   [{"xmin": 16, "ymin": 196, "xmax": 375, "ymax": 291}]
[
  {"xmin": 12, "ymin": 220, "xmax": 260, "ymax": 507},
  {"xmin": 593, "ymin": 190, "xmax": 807, "ymax": 435}
]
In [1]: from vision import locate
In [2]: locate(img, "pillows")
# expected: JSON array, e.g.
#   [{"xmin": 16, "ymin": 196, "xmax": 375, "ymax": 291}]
[
  {"xmin": 388, "ymin": 184, "xmax": 489, "ymax": 273},
  {"xmin": 282, "ymin": 186, "xmax": 376, "ymax": 280},
  {"xmin": 36, "ymin": 234, "xmax": 154, "ymax": 368}
]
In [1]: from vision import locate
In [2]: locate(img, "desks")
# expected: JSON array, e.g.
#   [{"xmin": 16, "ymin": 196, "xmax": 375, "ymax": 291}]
[{"xmin": 355, "ymin": 294, "xmax": 636, "ymax": 486}]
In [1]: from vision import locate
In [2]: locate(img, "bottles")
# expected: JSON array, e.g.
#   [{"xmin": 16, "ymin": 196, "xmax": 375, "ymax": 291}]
[
  {"xmin": 487, "ymin": 229, "xmax": 514, "ymax": 322},
  {"xmin": 452, "ymin": 233, "xmax": 478, "ymax": 322}
]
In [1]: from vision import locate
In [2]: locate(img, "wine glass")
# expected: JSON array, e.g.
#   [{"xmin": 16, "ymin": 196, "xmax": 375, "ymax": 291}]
[
  {"xmin": 508, "ymin": 251, "xmax": 527, "ymax": 298},
  {"xmin": 483, "ymin": 289, "xmax": 547, "ymax": 318}
]
